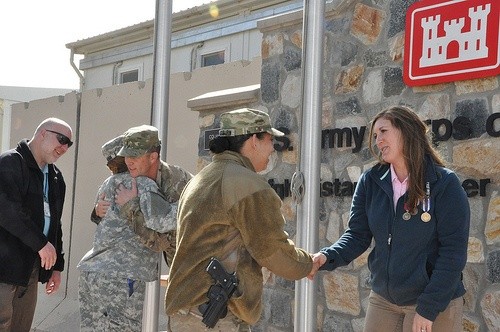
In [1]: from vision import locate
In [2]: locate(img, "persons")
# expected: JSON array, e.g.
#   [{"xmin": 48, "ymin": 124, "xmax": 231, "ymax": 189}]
[
  {"xmin": 0, "ymin": 117, "xmax": 74, "ymax": 332},
  {"xmin": 314, "ymin": 106, "xmax": 469, "ymax": 332},
  {"xmin": 165, "ymin": 108, "xmax": 319, "ymax": 332},
  {"xmin": 77, "ymin": 124, "xmax": 194, "ymax": 332}
]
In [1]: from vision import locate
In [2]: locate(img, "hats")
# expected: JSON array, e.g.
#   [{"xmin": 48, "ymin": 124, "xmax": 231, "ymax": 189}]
[
  {"xmin": 117, "ymin": 125, "xmax": 160, "ymax": 158},
  {"xmin": 101, "ymin": 135, "xmax": 124, "ymax": 161},
  {"xmin": 218, "ymin": 108, "xmax": 284, "ymax": 137}
]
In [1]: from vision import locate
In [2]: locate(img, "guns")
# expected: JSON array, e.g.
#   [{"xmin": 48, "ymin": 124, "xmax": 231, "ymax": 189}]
[{"xmin": 201, "ymin": 256, "xmax": 239, "ymax": 329}]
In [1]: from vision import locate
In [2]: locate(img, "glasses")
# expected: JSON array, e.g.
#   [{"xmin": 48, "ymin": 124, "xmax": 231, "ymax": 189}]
[{"xmin": 46, "ymin": 130, "xmax": 73, "ymax": 148}]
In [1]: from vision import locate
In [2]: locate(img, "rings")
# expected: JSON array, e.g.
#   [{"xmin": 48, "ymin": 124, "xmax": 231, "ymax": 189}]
[{"xmin": 421, "ymin": 329, "xmax": 425, "ymax": 332}]
